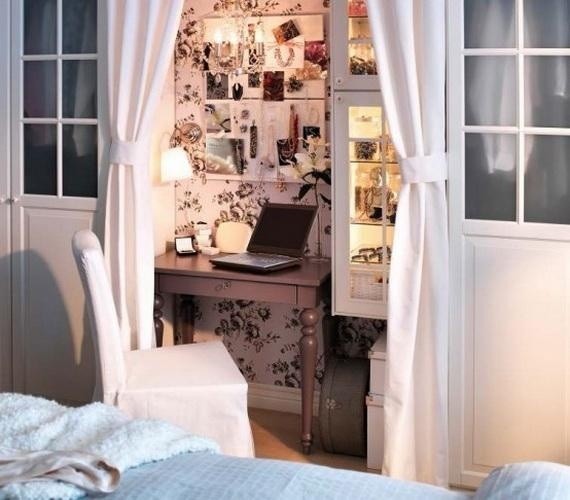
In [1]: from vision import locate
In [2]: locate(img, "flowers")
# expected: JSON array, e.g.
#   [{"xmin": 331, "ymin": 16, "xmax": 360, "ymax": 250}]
[{"xmin": 285, "ymin": 135, "xmax": 331, "ymax": 263}]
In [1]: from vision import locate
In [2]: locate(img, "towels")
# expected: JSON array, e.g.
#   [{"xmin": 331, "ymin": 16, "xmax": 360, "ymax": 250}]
[{"xmin": 1, "ymin": 393, "xmax": 221, "ymax": 499}]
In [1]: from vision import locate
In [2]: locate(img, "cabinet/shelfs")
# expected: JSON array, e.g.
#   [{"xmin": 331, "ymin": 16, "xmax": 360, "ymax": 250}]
[
  {"xmin": 328, "ymin": 0, "xmax": 383, "ymax": 91},
  {"xmin": 329, "ymin": 93, "xmax": 419, "ymax": 321}
]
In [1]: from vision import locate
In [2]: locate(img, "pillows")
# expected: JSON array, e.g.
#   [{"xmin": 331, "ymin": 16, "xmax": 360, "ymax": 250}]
[{"xmin": 474, "ymin": 460, "xmax": 570, "ymax": 500}]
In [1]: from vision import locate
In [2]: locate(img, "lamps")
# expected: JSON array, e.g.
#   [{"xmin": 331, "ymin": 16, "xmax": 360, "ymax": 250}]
[{"xmin": 160, "ymin": 124, "xmax": 201, "ymax": 182}]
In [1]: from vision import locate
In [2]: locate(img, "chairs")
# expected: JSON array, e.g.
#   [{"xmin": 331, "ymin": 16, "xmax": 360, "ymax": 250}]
[{"xmin": 72, "ymin": 229, "xmax": 253, "ymax": 458}]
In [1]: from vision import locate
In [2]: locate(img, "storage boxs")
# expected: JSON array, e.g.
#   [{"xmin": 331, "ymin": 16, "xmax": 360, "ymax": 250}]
[{"xmin": 365, "ymin": 330, "xmax": 387, "ymax": 471}]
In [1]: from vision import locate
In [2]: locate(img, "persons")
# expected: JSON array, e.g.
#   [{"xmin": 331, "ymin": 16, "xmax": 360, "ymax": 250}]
[{"xmin": 366, "ymin": 168, "xmax": 397, "ymax": 219}]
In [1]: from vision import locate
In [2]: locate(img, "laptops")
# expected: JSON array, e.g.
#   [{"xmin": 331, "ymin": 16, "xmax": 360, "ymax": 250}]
[{"xmin": 210, "ymin": 201, "xmax": 318, "ymax": 272}]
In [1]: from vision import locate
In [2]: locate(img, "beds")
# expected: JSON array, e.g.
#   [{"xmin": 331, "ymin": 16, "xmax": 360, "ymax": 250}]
[{"xmin": 1, "ymin": 394, "xmax": 474, "ymax": 500}]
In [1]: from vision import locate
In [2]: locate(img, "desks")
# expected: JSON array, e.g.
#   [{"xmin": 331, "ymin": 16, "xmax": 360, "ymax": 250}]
[{"xmin": 154, "ymin": 249, "xmax": 331, "ymax": 455}]
